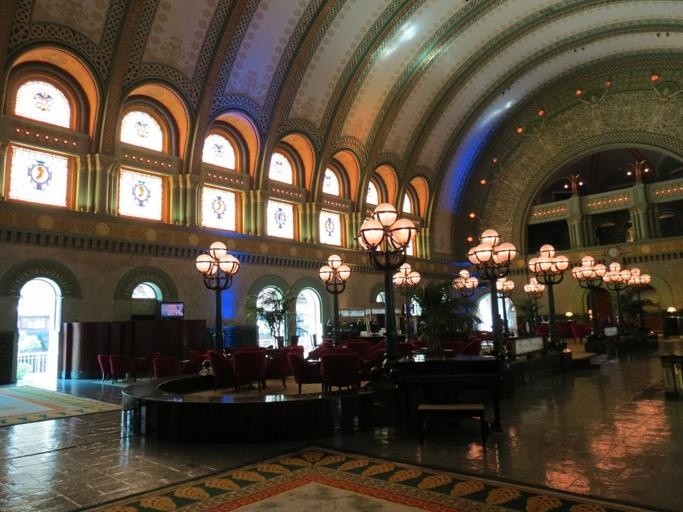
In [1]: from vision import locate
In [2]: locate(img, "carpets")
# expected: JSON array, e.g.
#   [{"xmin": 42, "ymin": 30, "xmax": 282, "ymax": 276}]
[
  {"xmin": 64, "ymin": 439, "xmax": 666, "ymax": 511},
  {"xmin": 0, "ymin": 386, "xmax": 122, "ymax": 426}
]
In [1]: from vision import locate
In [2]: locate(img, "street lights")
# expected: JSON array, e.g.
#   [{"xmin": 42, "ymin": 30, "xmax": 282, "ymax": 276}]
[
  {"xmin": 318, "ymin": 253, "xmax": 353, "ymax": 350},
  {"xmin": 571, "ymin": 255, "xmax": 655, "ymax": 352},
  {"xmin": 196, "ymin": 240, "xmax": 241, "ymax": 389},
  {"xmin": 449, "ymin": 227, "xmax": 519, "ymax": 369},
  {"xmin": 353, "ymin": 202, "xmax": 423, "ymax": 364},
  {"xmin": 525, "ymin": 239, "xmax": 557, "ymax": 349}
]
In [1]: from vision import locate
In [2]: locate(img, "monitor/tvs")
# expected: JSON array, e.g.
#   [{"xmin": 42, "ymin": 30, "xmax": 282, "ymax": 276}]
[{"xmin": 160, "ymin": 302, "xmax": 184, "ymax": 320}]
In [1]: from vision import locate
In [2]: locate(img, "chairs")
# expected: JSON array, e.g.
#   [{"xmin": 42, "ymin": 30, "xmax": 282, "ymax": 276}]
[
  {"xmin": 98, "ymin": 338, "xmax": 385, "ymax": 396},
  {"xmin": 411, "ymin": 337, "xmax": 483, "ymax": 355},
  {"xmin": 534, "ymin": 318, "xmax": 620, "ymax": 338}
]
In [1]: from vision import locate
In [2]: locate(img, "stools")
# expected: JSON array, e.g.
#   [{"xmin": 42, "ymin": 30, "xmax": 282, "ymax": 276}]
[{"xmin": 417, "ymin": 404, "xmax": 485, "ymax": 445}]
